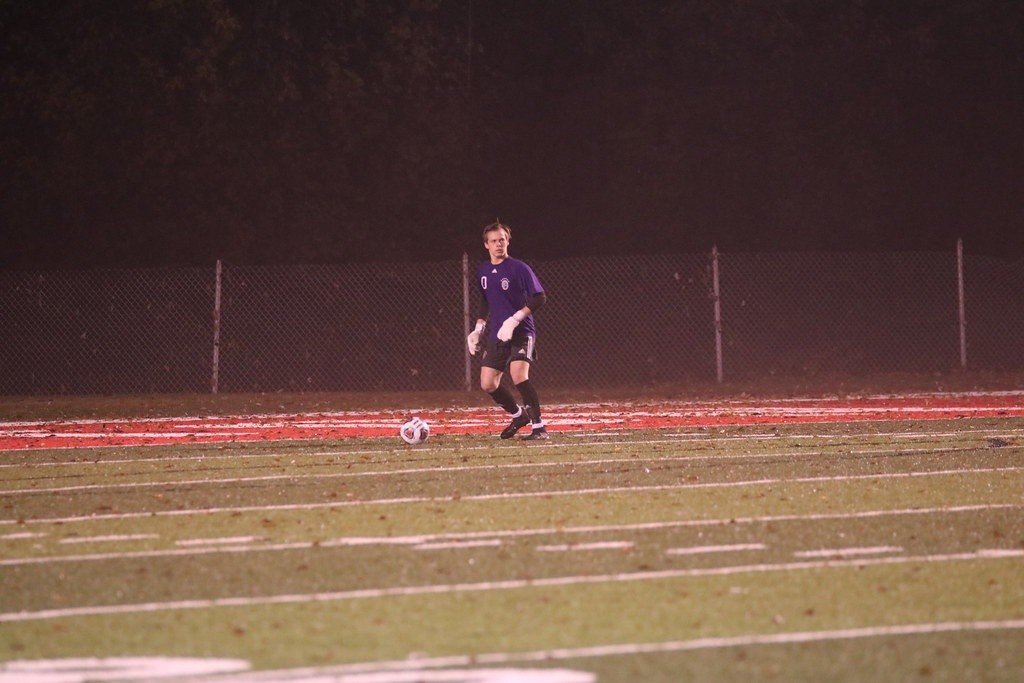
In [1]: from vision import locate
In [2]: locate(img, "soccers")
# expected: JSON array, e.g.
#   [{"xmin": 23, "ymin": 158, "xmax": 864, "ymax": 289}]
[{"xmin": 400, "ymin": 417, "xmax": 430, "ymax": 446}]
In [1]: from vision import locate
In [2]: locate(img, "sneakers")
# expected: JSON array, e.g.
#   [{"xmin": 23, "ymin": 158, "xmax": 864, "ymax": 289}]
[
  {"xmin": 501, "ymin": 407, "xmax": 530, "ymax": 439},
  {"xmin": 523, "ymin": 429, "xmax": 549, "ymax": 441}
]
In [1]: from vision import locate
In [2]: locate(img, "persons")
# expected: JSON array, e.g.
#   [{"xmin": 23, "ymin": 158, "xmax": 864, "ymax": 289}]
[{"xmin": 467, "ymin": 222, "xmax": 550, "ymax": 440}]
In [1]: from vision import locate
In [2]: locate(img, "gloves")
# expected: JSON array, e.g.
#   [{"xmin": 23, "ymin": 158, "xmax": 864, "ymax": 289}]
[
  {"xmin": 468, "ymin": 324, "xmax": 486, "ymax": 355},
  {"xmin": 496, "ymin": 316, "xmax": 520, "ymax": 342}
]
